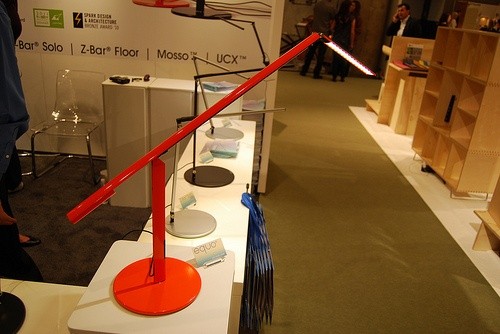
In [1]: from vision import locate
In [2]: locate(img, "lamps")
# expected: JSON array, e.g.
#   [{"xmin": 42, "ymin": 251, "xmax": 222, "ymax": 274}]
[{"xmin": 65, "ymin": 22, "xmax": 376, "ymax": 317}]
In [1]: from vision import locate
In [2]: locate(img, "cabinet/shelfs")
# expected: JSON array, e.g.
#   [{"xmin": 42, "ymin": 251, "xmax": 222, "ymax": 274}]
[{"xmin": 365, "ymin": 3, "xmax": 500, "ymax": 252}]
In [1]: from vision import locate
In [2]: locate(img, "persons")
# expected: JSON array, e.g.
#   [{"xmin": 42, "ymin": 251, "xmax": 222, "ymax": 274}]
[
  {"xmin": 385, "ymin": 3, "xmax": 417, "ymax": 60},
  {"xmin": 299, "ymin": 0, "xmax": 336, "ymax": 79},
  {"xmin": 0, "ymin": 0, "xmax": 45, "ymax": 284},
  {"xmin": 451, "ymin": 10, "xmax": 463, "ymax": 28},
  {"xmin": 331, "ymin": 0, "xmax": 357, "ymax": 82},
  {"xmin": 438, "ymin": 12, "xmax": 451, "ymax": 27},
  {"xmin": 350, "ymin": 0, "xmax": 362, "ymax": 40}
]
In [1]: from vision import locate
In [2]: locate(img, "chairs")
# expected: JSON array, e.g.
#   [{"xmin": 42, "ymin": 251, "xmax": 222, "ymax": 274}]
[{"xmin": 30, "ymin": 69, "xmax": 106, "ymax": 187}]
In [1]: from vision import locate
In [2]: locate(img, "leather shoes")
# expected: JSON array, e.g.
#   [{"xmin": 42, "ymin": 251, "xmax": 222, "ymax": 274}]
[{"xmin": 20, "ymin": 235, "xmax": 42, "ymax": 248}]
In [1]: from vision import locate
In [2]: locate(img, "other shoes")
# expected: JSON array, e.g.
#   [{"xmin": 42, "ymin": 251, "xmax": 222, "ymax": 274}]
[
  {"xmin": 332, "ymin": 76, "xmax": 337, "ymax": 81},
  {"xmin": 340, "ymin": 77, "xmax": 345, "ymax": 82},
  {"xmin": 299, "ymin": 71, "xmax": 306, "ymax": 76},
  {"xmin": 313, "ymin": 74, "xmax": 323, "ymax": 79}
]
